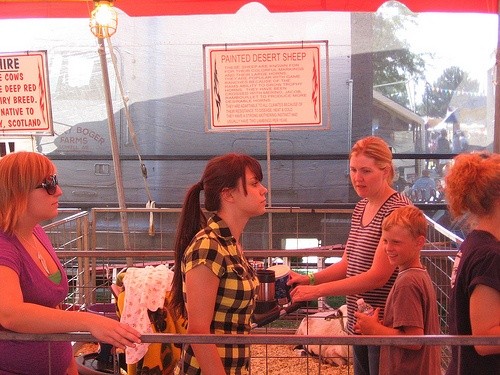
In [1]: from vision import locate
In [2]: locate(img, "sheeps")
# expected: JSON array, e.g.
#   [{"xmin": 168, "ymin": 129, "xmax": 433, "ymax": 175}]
[{"xmin": 293, "ymin": 305, "xmax": 354, "ymax": 365}]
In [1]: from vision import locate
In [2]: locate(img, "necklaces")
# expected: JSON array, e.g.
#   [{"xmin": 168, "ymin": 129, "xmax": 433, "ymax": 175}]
[{"xmin": 14, "ymin": 229, "xmax": 51, "ymax": 275}]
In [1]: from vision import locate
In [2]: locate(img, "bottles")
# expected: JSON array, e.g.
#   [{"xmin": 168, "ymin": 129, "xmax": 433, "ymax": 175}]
[{"xmin": 355, "ymin": 298, "xmax": 376, "ymax": 318}]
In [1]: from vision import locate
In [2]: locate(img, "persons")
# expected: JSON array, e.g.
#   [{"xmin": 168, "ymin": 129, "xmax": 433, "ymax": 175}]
[
  {"xmin": 442, "ymin": 153, "xmax": 500, "ymax": 375},
  {"xmin": 1, "ymin": 150, "xmax": 141, "ymax": 375},
  {"xmin": 390, "ymin": 128, "xmax": 469, "ymax": 203},
  {"xmin": 285, "ymin": 136, "xmax": 415, "ymax": 375},
  {"xmin": 354, "ymin": 205, "xmax": 441, "ymax": 375},
  {"xmin": 169, "ymin": 153, "xmax": 269, "ymax": 375}
]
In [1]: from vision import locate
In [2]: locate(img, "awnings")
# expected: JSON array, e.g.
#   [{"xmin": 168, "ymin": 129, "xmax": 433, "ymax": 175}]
[{"xmin": 444, "ymin": 96, "xmax": 487, "ymax": 124}]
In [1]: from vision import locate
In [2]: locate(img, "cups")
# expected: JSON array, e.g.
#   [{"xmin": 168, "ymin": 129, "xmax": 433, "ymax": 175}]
[
  {"xmin": 267, "ymin": 263, "xmax": 292, "ymax": 306},
  {"xmin": 255, "ymin": 269, "xmax": 275, "ymax": 314}
]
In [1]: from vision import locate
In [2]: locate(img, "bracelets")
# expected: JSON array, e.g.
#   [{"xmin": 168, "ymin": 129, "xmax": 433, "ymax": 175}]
[{"xmin": 308, "ymin": 273, "xmax": 315, "ymax": 286}]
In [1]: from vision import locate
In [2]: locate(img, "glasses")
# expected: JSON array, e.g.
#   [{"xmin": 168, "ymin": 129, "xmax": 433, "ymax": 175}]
[{"xmin": 35, "ymin": 174, "xmax": 58, "ymax": 195}]
